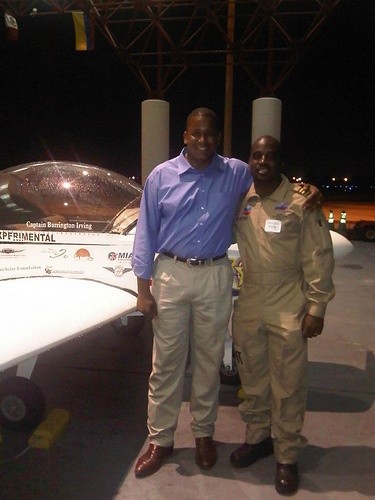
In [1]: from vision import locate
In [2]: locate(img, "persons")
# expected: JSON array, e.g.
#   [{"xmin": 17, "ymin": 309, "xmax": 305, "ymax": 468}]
[
  {"xmin": 131, "ymin": 108, "xmax": 324, "ymax": 478},
  {"xmin": 229, "ymin": 136, "xmax": 335, "ymax": 495}
]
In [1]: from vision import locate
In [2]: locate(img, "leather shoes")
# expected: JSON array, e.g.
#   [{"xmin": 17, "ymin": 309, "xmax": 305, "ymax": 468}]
[
  {"xmin": 230, "ymin": 435, "xmax": 274, "ymax": 468},
  {"xmin": 135, "ymin": 439, "xmax": 174, "ymax": 477},
  {"xmin": 195, "ymin": 436, "xmax": 218, "ymax": 471},
  {"xmin": 275, "ymin": 462, "xmax": 298, "ymax": 495}
]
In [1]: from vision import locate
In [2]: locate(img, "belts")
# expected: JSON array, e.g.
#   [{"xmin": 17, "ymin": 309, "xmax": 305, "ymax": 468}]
[{"xmin": 162, "ymin": 251, "xmax": 226, "ymax": 268}]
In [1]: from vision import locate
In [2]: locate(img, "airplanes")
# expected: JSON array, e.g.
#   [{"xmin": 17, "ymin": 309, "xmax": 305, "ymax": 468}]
[{"xmin": 1, "ymin": 159, "xmax": 356, "ymax": 432}]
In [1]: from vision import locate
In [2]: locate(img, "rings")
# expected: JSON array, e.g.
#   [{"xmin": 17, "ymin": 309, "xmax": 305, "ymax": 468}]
[{"xmin": 318, "ymin": 201, "xmax": 322, "ymax": 206}]
